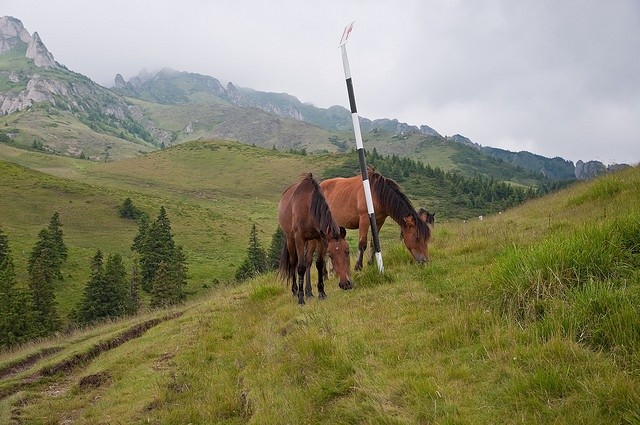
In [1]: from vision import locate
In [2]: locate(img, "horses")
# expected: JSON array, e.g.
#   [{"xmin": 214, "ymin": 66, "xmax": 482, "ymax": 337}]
[
  {"xmin": 275, "ymin": 172, "xmax": 353, "ymax": 308},
  {"xmin": 318, "ymin": 164, "xmax": 436, "ymax": 280}
]
